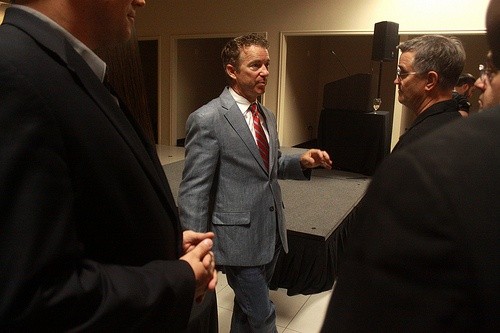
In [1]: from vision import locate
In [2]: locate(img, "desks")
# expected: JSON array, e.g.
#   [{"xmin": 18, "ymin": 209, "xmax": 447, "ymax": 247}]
[{"xmin": 313, "ymin": 110, "xmax": 391, "ymax": 177}]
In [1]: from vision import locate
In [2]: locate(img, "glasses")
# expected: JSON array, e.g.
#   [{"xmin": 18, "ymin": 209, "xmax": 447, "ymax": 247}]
[
  {"xmin": 396, "ymin": 68, "xmax": 432, "ymax": 81},
  {"xmin": 479, "ymin": 64, "xmax": 500, "ymax": 82}
]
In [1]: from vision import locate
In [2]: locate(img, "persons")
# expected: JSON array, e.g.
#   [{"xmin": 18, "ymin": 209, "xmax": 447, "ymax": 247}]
[
  {"xmin": 0, "ymin": 0, "xmax": 218, "ymax": 333},
  {"xmin": 319, "ymin": 0, "xmax": 500, "ymax": 333},
  {"xmin": 177, "ymin": 33, "xmax": 333, "ymax": 333}
]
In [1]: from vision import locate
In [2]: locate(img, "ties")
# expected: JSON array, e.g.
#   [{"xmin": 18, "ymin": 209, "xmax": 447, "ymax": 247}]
[{"xmin": 249, "ymin": 102, "xmax": 270, "ymax": 169}]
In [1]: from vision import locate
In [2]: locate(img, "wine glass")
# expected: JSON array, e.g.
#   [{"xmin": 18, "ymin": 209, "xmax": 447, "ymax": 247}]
[{"xmin": 372, "ymin": 98, "xmax": 381, "ymax": 114}]
